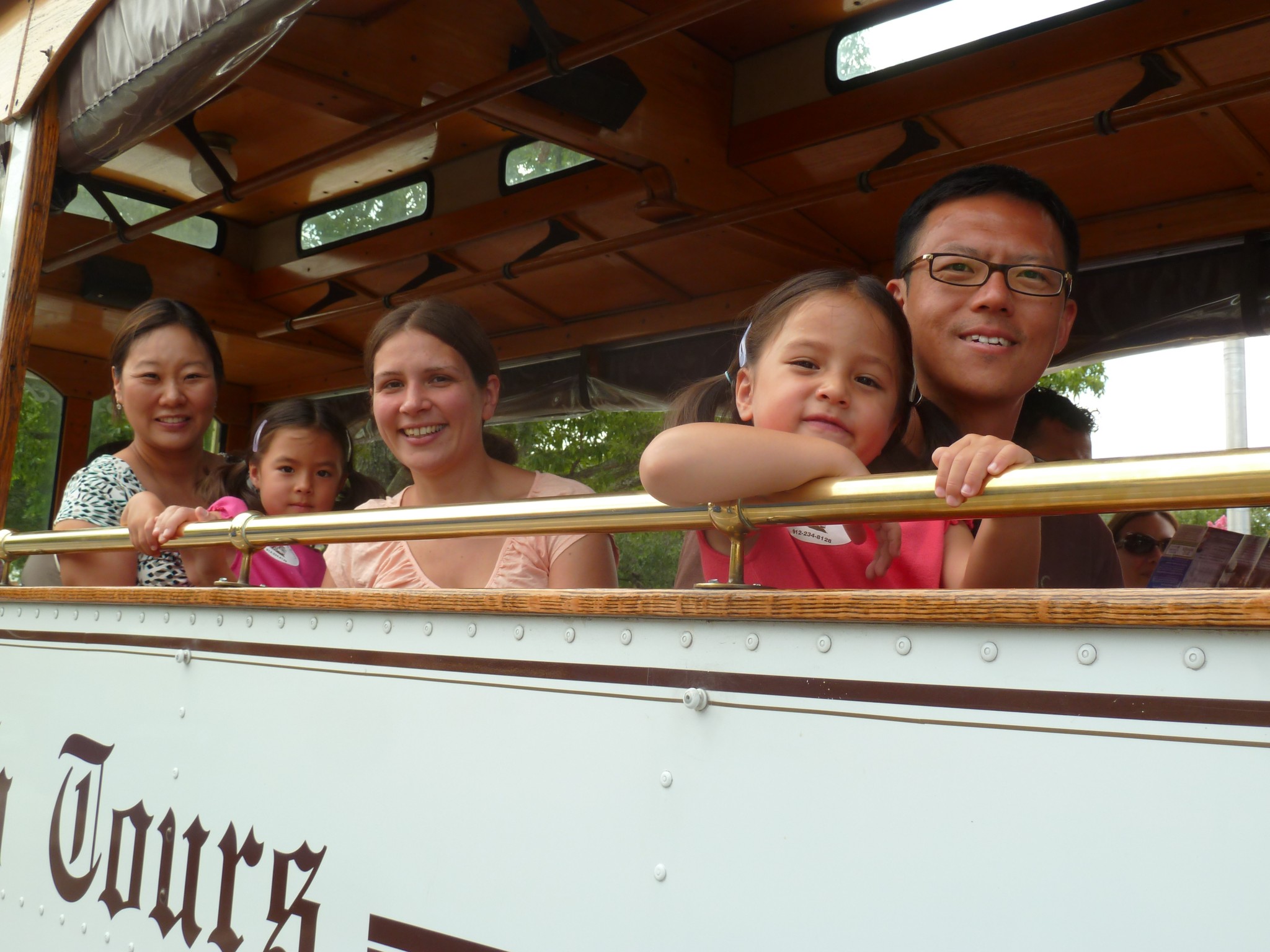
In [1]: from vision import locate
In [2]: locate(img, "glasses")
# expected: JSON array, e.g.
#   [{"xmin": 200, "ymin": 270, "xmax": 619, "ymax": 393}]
[
  {"xmin": 899, "ymin": 252, "xmax": 1072, "ymax": 298},
  {"xmin": 1115, "ymin": 531, "xmax": 1173, "ymax": 555}
]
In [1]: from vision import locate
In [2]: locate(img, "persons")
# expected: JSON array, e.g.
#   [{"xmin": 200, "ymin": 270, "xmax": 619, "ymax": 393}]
[
  {"xmin": 671, "ymin": 164, "xmax": 1126, "ymax": 587},
  {"xmin": 19, "ymin": 438, "xmax": 135, "ymax": 586},
  {"xmin": 151, "ymin": 297, "xmax": 623, "ymax": 593},
  {"xmin": 1105, "ymin": 509, "xmax": 1180, "ymax": 589},
  {"xmin": 49, "ymin": 295, "xmax": 260, "ymax": 586},
  {"xmin": 1011, "ymin": 382, "xmax": 1098, "ymax": 463},
  {"xmin": 117, "ymin": 395, "xmax": 387, "ymax": 590},
  {"xmin": 636, "ymin": 268, "xmax": 1046, "ymax": 590}
]
